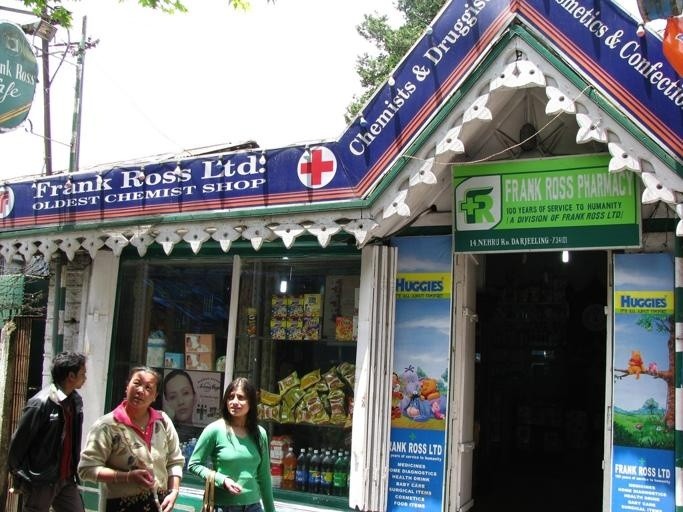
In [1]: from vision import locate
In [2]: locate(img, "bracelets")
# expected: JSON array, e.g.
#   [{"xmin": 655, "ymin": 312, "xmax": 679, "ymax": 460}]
[
  {"xmin": 126, "ymin": 470, "xmax": 130, "ymax": 484},
  {"xmin": 115, "ymin": 467, "xmax": 120, "ymax": 485}
]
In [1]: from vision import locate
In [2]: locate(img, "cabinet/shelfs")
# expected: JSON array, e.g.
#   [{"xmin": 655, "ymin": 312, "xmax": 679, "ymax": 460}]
[{"xmin": 262, "ymin": 337, "xmax": 356, "ymax": 498}]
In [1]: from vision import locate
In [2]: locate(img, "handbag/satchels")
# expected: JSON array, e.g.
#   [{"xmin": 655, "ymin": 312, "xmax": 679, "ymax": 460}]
[{"xmin": 105, "ymin": 490, "xmax": 162, "ymax": 512}]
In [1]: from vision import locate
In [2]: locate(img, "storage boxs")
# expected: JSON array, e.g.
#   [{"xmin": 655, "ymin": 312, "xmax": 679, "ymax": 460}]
[{"xmin": 321, "ymin": 275, "xmax": 359, "ymax": 338}]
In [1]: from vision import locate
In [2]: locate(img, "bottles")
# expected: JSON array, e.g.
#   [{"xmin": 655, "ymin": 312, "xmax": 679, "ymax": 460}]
[{"xmin": 269, "ymin": 437, "xmax": 350, "ymax": 497}]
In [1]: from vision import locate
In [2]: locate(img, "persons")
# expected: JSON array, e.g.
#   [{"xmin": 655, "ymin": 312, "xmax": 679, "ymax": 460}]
[
  {"xmin": 164, "ymin": 369, "xmax": 198, "ymax": 423},
  {"xmin": 186, "ymin": 377, "xmax": 278, "ymax": 512},
  {"xmin": 78, "ymin": 365, "xmax": 186, "ymax": 512},
  {"xmin": 6, "ymin": 352, "xmax": 87, "ymax": 511}
]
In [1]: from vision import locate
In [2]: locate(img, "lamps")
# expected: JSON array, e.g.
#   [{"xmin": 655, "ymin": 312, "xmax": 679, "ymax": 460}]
[
  {"xmin": 31, "ymin": 143, "xmax": 311, "ymax": 190},
  {"xmin": 359, "ymin": 1, "xmax": 650, "ymax": 127},
  {"xmin": 279, "ymin": 276, "xmax": 287, "ymax": 293}
]
